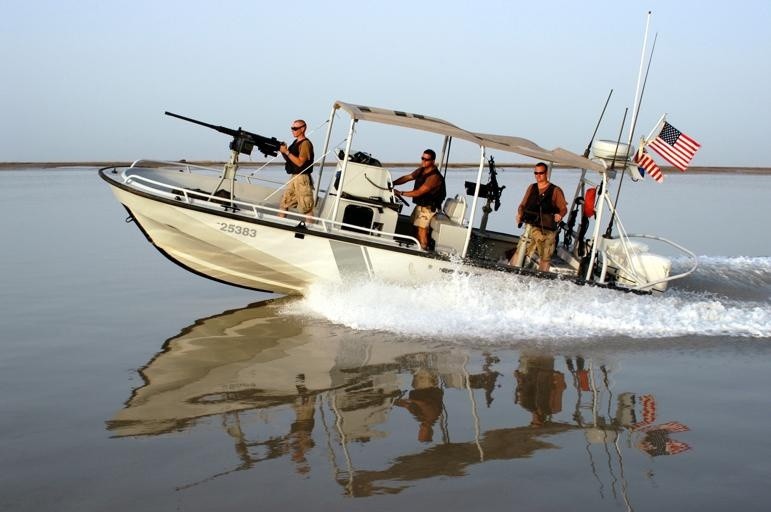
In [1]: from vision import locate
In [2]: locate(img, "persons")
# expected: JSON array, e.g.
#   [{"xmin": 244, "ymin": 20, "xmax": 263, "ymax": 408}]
[
  {"xmin": 527, "ymin": 365, "xmax": 551, "ymax": 432},
  {"xmin": 515, "ymin": 162, "xmax": 568, "ymax": 272},
  {"xmin": 289, "ymin": 393, "xmax": 314, "ymax": 471},
  {"xmin": 394, "ymin": 368, "xmax": 443, "ymax": 441},
  {"xmin": 391, "ymin": 149, "xmax": 446, "ymax": 252},
  {"xmin": 277, "ymin": 118, "xmax": 315, "ymax": 222}
]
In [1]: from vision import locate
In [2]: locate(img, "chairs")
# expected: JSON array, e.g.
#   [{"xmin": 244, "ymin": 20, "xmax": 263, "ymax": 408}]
[{"xmin": 430, "ymin": 193, "xmax": 463, "ymax": 233}]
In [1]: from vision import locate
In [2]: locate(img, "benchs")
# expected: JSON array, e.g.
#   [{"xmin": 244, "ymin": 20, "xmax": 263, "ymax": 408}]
[{"xmin": 529, "ymin": 248, "xmax": 577, "ymax": 275}]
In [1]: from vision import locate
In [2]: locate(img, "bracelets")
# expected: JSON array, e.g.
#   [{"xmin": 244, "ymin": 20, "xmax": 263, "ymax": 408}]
[
  {"xmin": 285, "ymin": 150, "xmax": 290, "ymax": 156},
  {"xmin": 401, "ymin": 192, "xmax": 404, "ymax": 195}
]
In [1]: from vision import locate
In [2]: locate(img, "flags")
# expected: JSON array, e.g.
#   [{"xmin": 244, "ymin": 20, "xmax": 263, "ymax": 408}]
[
  {"xmin": 633, "ymin": 152, "xmax": 663, "ymax": 184},
  {"xmin": 638, "ymin": 395, "xmax": 657, "ymax": 421},
  {"xmin": 640, "ymin": 422, "xmax": 688, "ymax": 437},
  {"xmin": 638, "ymin": 435, "xmax": 694, "ymax": 456},
  {"xmin": 648, "ymin": 121, "xmax": 702, "ymax": 174}
]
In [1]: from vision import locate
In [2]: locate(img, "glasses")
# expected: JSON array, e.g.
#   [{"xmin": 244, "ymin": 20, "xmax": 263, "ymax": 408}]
[
  {"xmin": 535, "ymin": 172, "xmax": 544, "ymax": 174},
  {"xmin": 421, "ymin": 157, "xmax": 431, "ymax": 161},
  {"xmin": 291, "ymin": 124, "xmax": 305, "ymax": 131}
]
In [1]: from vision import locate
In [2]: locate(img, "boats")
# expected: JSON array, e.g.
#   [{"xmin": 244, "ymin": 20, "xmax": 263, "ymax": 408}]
[
  {"xmin": 95, "ymin": 10, "xmax": 700, "ymax": 296},
  {"xmin": 106, "ymin": 298, "xmax": 637, "ymax": 502}
]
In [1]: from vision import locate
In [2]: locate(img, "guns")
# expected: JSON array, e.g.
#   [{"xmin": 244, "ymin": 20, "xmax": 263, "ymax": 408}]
[
  {"xmin": 170, "ymin": 436, "xmax": 286, "ymax": 489},
  {"xmin": 481, "ymin": 352, "xmax": 499, "ymax": 406},
  {"xmin": 163, "ymin": 110, "xmax": 285, "ymax": 159},
  {"xmin": 489, "ymin": 154, "xmax": 504, "ymax": 212}
]
